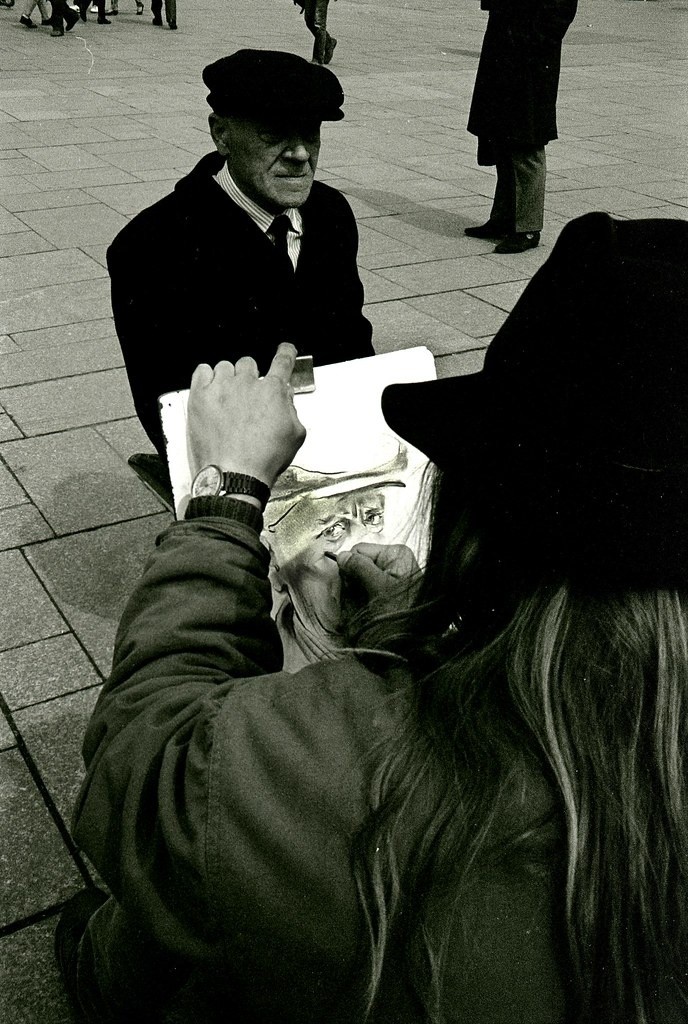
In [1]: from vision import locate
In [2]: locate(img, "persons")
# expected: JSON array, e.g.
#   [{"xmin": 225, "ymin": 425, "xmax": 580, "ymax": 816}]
[
  {"xmin": 294, "ymin": 0, "xmax": 337, "ymax": 66},
  {"xmin": 151, "ymin": 0, "xmax": 177, "ymax": 30},
  {"xmin": 104, "ymin": 48, "xmax": 377, "ymax": 482},
  {"xmin": 464, "ymin": 0, "xmax": 576, "ymax": 253},
  {"xmin": 52, "ymin": 210, "xmax": 688, "ymax": 1024},
  {"xmin": 20, "ymin": 0, "xmax": 144, "ymax": 37}
]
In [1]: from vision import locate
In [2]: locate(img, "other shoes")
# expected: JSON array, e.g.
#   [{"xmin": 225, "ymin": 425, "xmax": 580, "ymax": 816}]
[
  {"xmin": 51, "ymin": 30, "xmax": 63, "ymax": 36},
  {"xmin": 42, "ymin": 18, "xmax": 52, "ymax": 25},
  {"xmin": 310, "ymin": 56, "xmax": 323, "ymax": 66},
  {"xmin": 169, "ymin": 18, "xmax": 178, "ymax": 29},
  {"xmin": 465, "ymin": 214, "xmax": 518, "ymax": 238},
  {"xmin": 153, "ymin": 19, "xmax": 163, "ymax": 26},
  {"xmin": 494, "ymin": 231, "xmax": 539, "ymax": 254},
  {"xmin": 70, "ymin": 5, "xmax": 80, "ymax": 13},
  {"xmin": 66, "ymin": 15, "xmax": 79, "ymax": 31},
  {"xmin": 323, "ymin": 38, "xmax": 337, "ymax": 64},
  {"xmin": 105, "ymin": 8, "xmax": 119, "ymax": 16},
  {"xmin": 90, "ymin": 5, "xmax": 99, "ymax": 12},
  {"xmin": 137, "ymin": 5, "xmax": 143, "ymax": 14},
  {"xmin": 20, "ymin": 16, "xmax": 37, "ymax": 28},
  {"xmin": 80, "ymin": 4, "xmax": 86, "ymax": 22},
  {"xmin": 98, "ymin": 17, "xmax": 112, "ymax": 24}
]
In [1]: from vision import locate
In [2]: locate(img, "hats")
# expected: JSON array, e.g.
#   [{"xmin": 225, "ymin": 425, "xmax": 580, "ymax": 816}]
[
  {"xmin": 202, "ymin": 50, "xmax": 345, "ymax": 123},
  {"xmin": 381, "ymin": 210, "xmax": 687, "ymax": 543}
]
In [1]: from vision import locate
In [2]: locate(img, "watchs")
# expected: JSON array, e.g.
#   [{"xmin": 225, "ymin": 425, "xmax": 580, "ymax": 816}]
[{"xmin": 191, "ymin": 464, "xmax": 270, "ymax": 511}]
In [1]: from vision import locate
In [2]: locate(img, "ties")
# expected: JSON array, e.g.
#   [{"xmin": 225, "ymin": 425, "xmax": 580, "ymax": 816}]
[{"xmin": 267, "ymin": 215, "xmax": 299, "ymax": 274}]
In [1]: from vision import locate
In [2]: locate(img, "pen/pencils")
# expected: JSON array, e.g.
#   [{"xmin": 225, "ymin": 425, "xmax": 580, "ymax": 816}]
[{"xmin": 323, "ymin": 548, "xmax": 340, "ymax": 563}]
